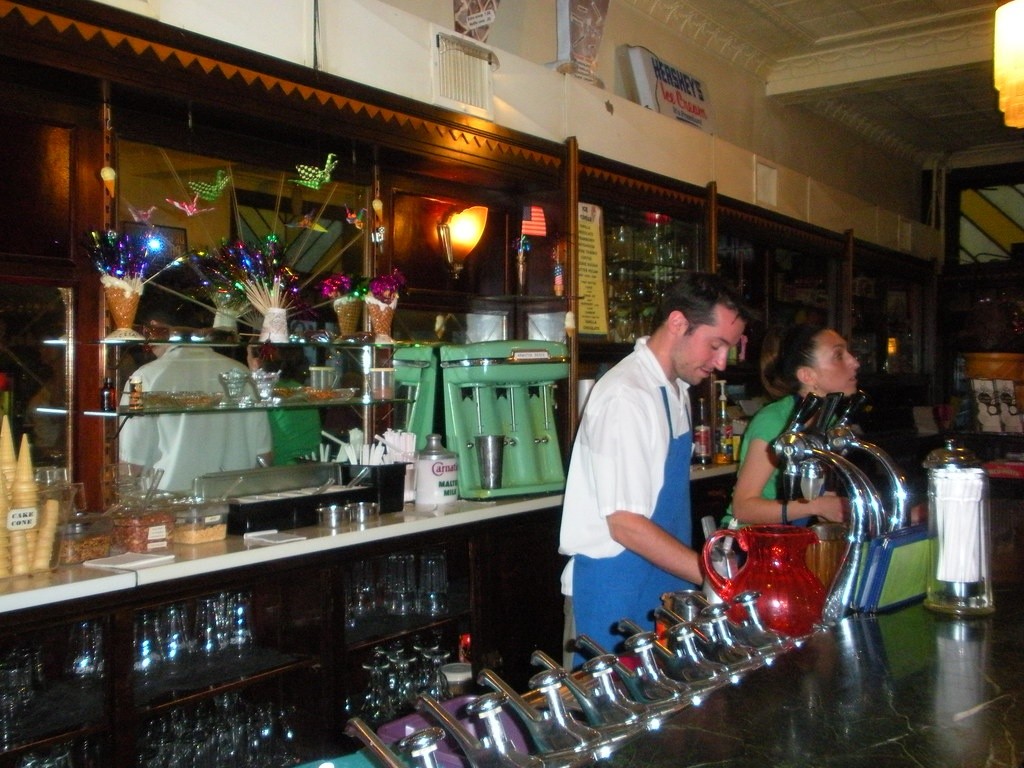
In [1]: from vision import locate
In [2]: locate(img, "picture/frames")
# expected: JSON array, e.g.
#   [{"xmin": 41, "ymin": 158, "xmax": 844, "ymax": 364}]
[{"xmin": 123, "ymin": 221, "xmax": 188, "ymax": 257}]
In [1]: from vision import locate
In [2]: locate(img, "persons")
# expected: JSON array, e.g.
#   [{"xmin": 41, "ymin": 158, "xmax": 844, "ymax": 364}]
[
  {"xmin": 116, "ymin": 306, "xmax": 274, "ymax": 500},
  {"xmin": 247, "ymin": 343, "xmax": 324, "ymax": 471},
  {"xmin": 559, "ymin": 268, "xmax": 748, "ymax": 674},
  {"xmin": 719, "ymin": 321, "xmax": 932, "ymax": 534}
]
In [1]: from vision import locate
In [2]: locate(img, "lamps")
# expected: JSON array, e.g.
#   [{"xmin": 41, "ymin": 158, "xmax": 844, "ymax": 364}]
[
  {"xmin": 994, "ymin": 0, "xmax": 1024, "ymax": 128},
  {"xmin": 437, "ymin": 206, "xmax": 489, "ymax": 282}
]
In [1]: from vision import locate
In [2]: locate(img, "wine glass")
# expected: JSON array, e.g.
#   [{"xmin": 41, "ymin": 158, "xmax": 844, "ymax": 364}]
[{"xmin": 345, "ymin": 632, "xmax": 451, "ymax": 726}]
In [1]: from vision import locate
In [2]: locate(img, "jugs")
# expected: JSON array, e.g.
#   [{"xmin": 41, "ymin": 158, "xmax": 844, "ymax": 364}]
[{"xmin": 699, "ymin": 523, "xmax": 827, "ymax": 638}]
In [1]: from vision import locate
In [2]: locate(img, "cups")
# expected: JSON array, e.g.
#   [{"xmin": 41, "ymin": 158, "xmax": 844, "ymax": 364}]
[
  {"xmin": 366, "ymin": 367, "xmax": 396, "ymax": 400},
  {"xmin": 474, "ymin": 435, "xmax": 506, "ymax": 489},
  {"xmin": 131, "ymin": 594, "xmax": 252, "ymax": 676},
  {"xmin": 139, "ymin": 692, "xmax": 304, "ymax": 768},
  {"xmin": 346, "ymin": 553, "xmax": 450, "ymax": 625},
  {"xmin": 309, "ymin": 365, "xmax": 340, "ymax": 391},
  {"xmin": 606, "ymin": 226, "xmax": 690, "ymax": 344},
  {"xmin": 317, "ymin": 506, "xmax": 352, "ymax": 528},
  {"xmin": 347, "ymin": 502, "xmax": 380, "ymax": 520},
  {"xmin": 0, "ymin": 619, "xmax": 108, "ymax": 768}
]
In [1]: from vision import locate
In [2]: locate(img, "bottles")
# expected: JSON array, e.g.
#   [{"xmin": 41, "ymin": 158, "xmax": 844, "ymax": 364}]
[
  {"xmin": 101, "ymin": 377, "xmax": 116, "ymax": 410},
  {"xmin": 696, "ymin": 397, "xmax": 713, "ymax": 465},
  {"xmin": 128, "ymin": 376, "xmax": 144, "ymax": 410}
]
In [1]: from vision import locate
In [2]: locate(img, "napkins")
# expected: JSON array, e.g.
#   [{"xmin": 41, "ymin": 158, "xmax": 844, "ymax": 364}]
[
  {"xmin": 244, "ymin": 530, "xmax": 306, "ymax": 543},
  {"xmin": 84, "ymin": 552, "xmax": 176, "ymax": 567}
]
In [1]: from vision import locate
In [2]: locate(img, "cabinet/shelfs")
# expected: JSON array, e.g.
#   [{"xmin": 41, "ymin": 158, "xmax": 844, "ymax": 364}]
[
  {"xmin": 0, "ymin": 534, "xmax": 479, "ymax": 768},
  {"xmin": 35, "ymin": 335, "xmax": 416, "ymax": 482},
  {"xmin": 0, "ymin": 0, "xmax": 936, "ymax": 611}
]
